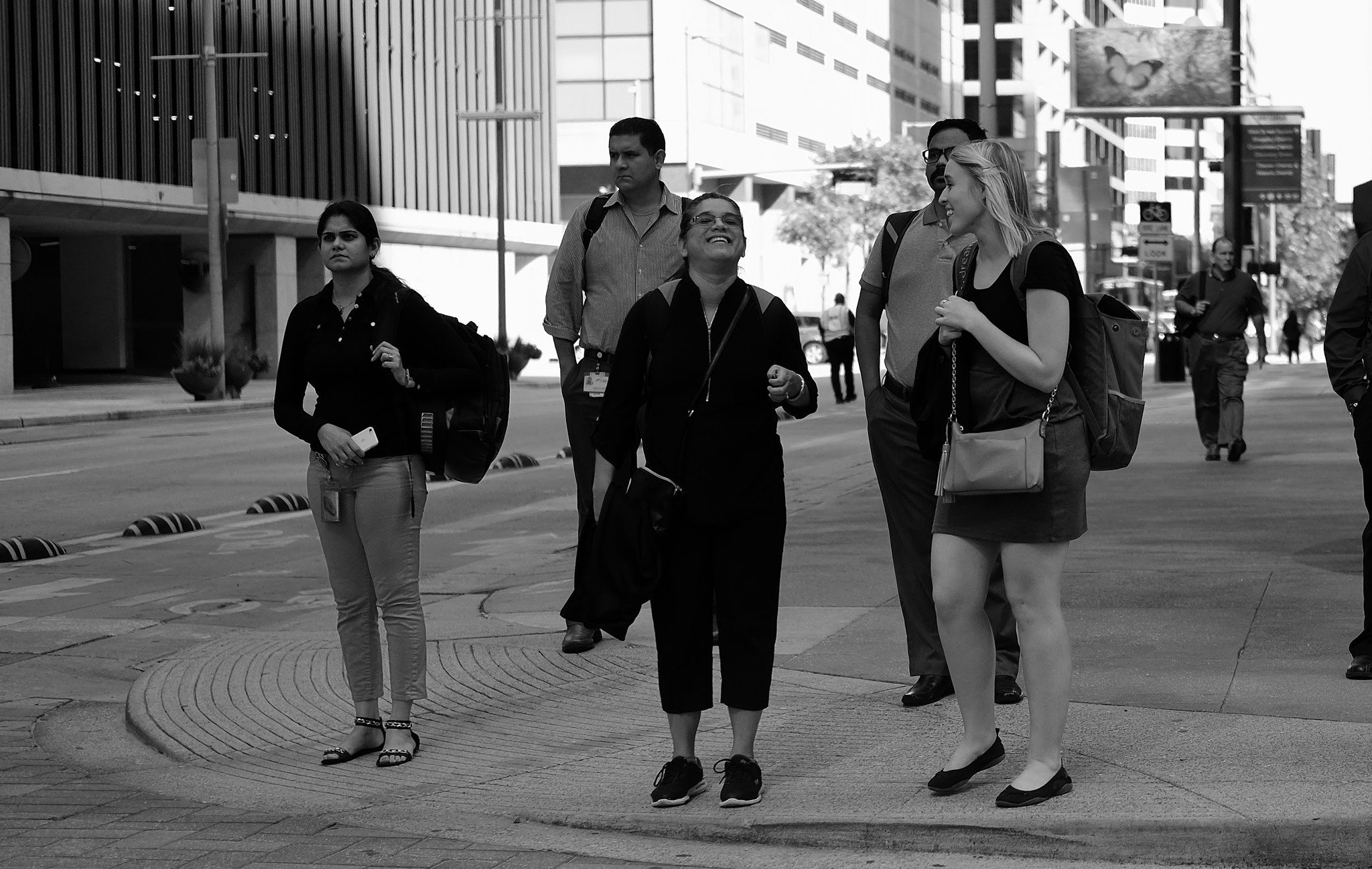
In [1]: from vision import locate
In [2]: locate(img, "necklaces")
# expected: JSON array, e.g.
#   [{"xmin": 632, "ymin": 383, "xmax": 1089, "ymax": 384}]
[
  {"xmin": 630, "ymin": 206, "xmax": 656, "ymax": 217},
  {"xmin": 333, "ymin": 289, "xmax": 356, "ymax": 315}
]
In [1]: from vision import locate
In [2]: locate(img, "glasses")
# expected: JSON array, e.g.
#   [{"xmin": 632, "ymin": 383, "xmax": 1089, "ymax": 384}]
[
  {"xmin": 685, "ymin": 214, "xmax": 744, "ymax": 227},
  {"xmin": 923, "ymin": 146, "xmax": 956, "ymax": 164}
]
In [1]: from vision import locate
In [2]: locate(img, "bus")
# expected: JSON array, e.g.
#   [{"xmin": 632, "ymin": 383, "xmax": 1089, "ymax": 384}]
[{"xmin": 1092, "ymin": 275, "xmax": 1166, "ymax": 353}]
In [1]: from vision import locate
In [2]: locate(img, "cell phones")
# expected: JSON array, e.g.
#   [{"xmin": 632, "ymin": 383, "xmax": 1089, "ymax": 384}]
[{"xmin": 336, "ymin": 426, "xmax": 378, "ymax": 466}]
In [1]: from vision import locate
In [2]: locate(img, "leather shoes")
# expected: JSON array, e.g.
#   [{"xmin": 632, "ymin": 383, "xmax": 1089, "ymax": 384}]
[
  {"xmin": 995, "ymin": 676, "xmax": 1023, "ymax": 703},
  {"xmin": 1346, "ymin": 655, "xmax": 1372, "ymax": 678},
  {"xmin": 902, "ymin": 675, "xmax": 953, "ymax": 706},
  {"xmin": 562, "ymin": 620, "xmax": 602, "ymax": 653}
]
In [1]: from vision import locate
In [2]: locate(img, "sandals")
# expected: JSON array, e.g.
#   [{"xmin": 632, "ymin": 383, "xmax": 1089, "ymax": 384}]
[
  {"xmin": 376, "ymin": 720, "xmax": 420, "ymax": 766},
  {"xmin": 321, "ymin": 717, "xmax": 384, "ymax": 764}
]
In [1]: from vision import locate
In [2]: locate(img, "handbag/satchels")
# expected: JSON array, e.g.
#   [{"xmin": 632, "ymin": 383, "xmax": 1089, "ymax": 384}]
[
  {"xmin": 933, "ymin": 418, "xmax": 1047, "ymax": 503},
  {"xmin": 1173, "ymin": 270, "xmax": 1207, "ymax": 337}
]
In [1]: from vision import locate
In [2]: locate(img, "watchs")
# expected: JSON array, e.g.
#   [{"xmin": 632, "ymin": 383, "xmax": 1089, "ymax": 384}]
[
  {"xmin": 406, "ymin": 369, "xmax": 415, "ymax": 388},
  {"xmin": 788, "ymin": 374, "xmax": 807, "ymax": 403}
]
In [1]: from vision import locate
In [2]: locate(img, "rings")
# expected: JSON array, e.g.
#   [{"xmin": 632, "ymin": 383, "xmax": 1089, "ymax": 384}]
[
  {"xmin": 942, "ymin": 300, "xmax": 947, "ymax": 306},
  {"xmin": 389, "ymin": 355, "xmax": 393, "ymax": 360},
  {"xmin": 786, "ymin": 391, "xmax": 791, "ymax": 401}
]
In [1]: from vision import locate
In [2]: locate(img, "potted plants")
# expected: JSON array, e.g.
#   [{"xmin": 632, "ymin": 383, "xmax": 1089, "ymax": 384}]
[
  {"xmin": 170, "ymin": 328, "xmax": 269, "ymax": 402},
  {"xmin": 495, "ymin": 338, "xmax": 542, "ymax": 381}
]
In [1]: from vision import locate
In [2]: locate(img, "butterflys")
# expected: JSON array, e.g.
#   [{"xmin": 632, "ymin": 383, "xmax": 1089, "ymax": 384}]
[{"xmin": 1104, "ymin": 45, "xmax": 1163, "ymax": 90}]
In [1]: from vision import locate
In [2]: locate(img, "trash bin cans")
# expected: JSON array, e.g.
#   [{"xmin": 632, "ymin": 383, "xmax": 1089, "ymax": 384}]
[{"xmin": 1158, "ymin": 333, "xmax": 1185, "ymax": 383}]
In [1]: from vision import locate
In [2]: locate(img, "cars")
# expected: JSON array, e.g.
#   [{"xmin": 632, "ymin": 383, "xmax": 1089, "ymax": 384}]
[{"xmin": 792, "ymin": 311, "xmax": 830, "ymax": 365}]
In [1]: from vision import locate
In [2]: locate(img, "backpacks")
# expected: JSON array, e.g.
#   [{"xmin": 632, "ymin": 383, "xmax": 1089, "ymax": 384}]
[
  {"xmin": 957, "ymin": 231, "xmax": 1149, "ymax": 471},
  {"xmin": 378, "ymin": 284, "xmax": 511, "ymax": 485}
]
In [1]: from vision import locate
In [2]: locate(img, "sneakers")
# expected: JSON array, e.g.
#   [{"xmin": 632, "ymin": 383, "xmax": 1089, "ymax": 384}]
[
  {"xmin": 651, "ymin": 756, "xmax": 707, "ymax": 806},
  {"xmin": 713, "ymin": 754, "xmax": 766, "ymax": 806}
]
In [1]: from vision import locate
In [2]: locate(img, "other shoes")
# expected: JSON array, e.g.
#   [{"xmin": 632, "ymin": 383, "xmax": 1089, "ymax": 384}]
[
  {"xmin": 1206, "ymin": 443, "xmax": 1222, "ymax": 460},
  {"xmin": 928, "ymin": 728, "xmax": 1006, "ymax": 793},
  {"xmin": 845, "ymin": 394, "xmax": 856, "ymax": 402},
  {"xmin": 836, "ymin": 398, "xmax": 844, "ymax": 404},
  {"xmin": 1227, "ymin": 439, "xmax": 1246, "ymax": 462},
  {"xmin": 995, "ymin": 768, "xmax": 1073, "ymax": 806}
]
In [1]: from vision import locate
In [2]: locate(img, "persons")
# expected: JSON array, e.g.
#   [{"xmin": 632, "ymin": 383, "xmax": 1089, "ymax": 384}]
[
  {"xmin": 854, "ymin": 118, "xmax": 1024, "ymax": 706},
  {"xmin": 591, "ymin": 192, "xmax": 818, "ymax": 807},
  {"xmin": 817, "ymin": 293, "xmax": 857, "ymax": 403},
  {"xmin": 274, "ymin": 200, "xmax": 434, "ymax": 768},
  {"xmin": 1282, "ymin": 296, "xmax": 1334, "ymax": 365},
  {"xmin": 1323, "ymin": 228, "xmax": 1372, "ymax": 680},
  {"xmin": 543, "ymin": 118, "xmax": 695, "ymax": 654},
  {"xmin": 1174, "ymin": 239, "xmax": 1268, "ymax": 463},
  {"xmin": 926, "ymin": 140, "xmax": 1091, "ymax": 807}
]
